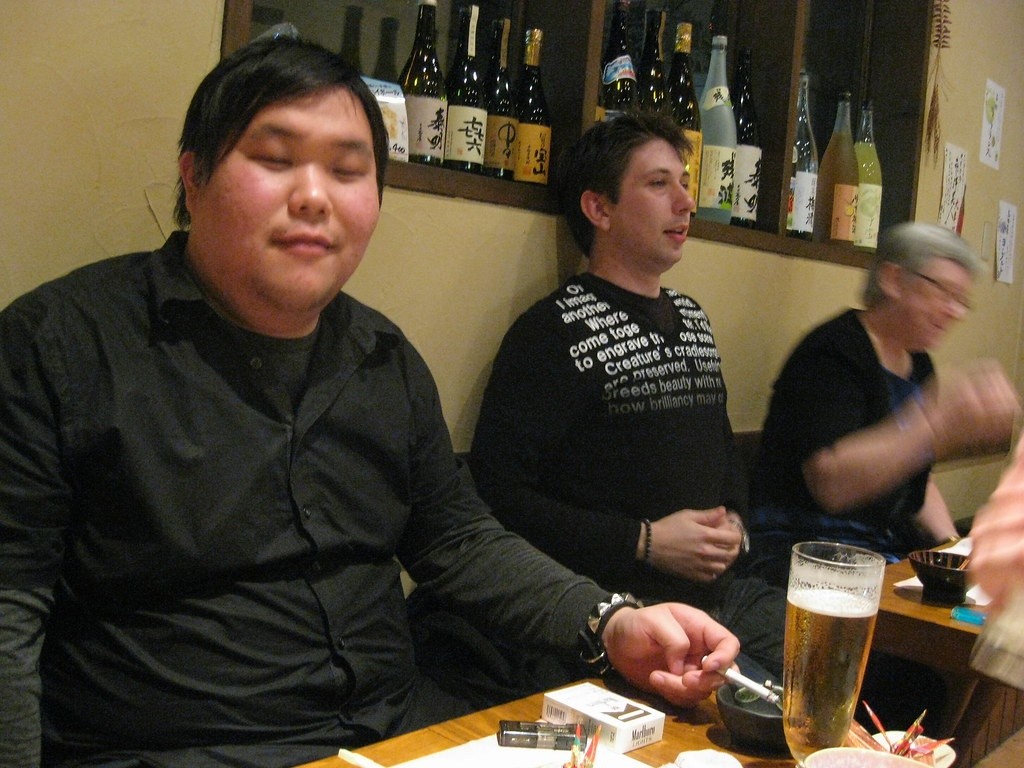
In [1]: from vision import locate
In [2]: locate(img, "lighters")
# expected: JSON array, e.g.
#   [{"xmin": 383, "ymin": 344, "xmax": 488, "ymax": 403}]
[{"xmin": 498, "ymin": 720, "xmax": 588, "ymax": 751}]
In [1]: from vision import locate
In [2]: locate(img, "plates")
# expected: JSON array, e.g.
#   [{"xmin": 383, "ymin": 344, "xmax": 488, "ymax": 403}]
[{"xmin": 872, "ymin": 731, "xmax": 956, "ymax": 768}]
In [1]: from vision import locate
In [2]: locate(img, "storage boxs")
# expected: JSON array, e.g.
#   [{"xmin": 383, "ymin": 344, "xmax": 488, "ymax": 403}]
[{"xmin": 541, "ymin": 681, "xmax": 666, "ymax": 754}]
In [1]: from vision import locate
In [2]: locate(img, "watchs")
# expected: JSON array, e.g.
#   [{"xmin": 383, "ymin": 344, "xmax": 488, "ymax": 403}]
[{"xmin": 728, "ymin": 517, "xmax": 751, "ymax": 555}]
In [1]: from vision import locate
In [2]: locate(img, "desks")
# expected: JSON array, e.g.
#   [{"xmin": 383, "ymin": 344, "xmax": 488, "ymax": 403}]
[
  {"xmin": 297, "ymin": 663, "xmax": 797, "ymax": 768},
  {"xmin": 867, "ymin": 540, "xmax": 1024, "ymax": 768}
]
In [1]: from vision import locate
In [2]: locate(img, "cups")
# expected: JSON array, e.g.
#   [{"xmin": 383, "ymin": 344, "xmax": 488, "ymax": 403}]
[{"xmin": 782, "ymin": 541, "xmax": 885, "ymax": 768}]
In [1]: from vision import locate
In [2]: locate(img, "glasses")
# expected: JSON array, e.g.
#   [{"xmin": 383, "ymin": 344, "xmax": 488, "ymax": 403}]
[{"xmin": 912, "ymin": 268, "xmax": 973, "ymax": 312}]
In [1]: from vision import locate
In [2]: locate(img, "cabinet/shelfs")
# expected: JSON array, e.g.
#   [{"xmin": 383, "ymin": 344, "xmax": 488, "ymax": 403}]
[{"xmin": 220, "ymin": 0, "xmax": 939, "ymax": 270}]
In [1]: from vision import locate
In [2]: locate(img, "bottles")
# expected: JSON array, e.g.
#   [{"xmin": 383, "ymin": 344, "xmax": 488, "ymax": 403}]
[
  {"xmin": 793, "ymin": 73, "xmax": 818, "ymax": 241},
  {"xmin": 731, "ymin": 47, "xmax": 762, "ymax": 230},
  {"xmin": 513, "ymin": 28, "xmax": 551, "ymax": 186},
  {"xmin": 483, "ymin": 17, "xmax": 519, "ymax": 181},
  {"xmin": 638, "ymin": 11, "xmax": 668, "ymax": 122},
  {"xmin": 595, "ymin": 68, "xmax": 605, "ymax": 123},
  {"xmin": 696, "ymin": 36, "xmax": 737, "ymax": 224},
  {"xmin": 603, "ymin": 0, "xmax": 641, "ymax": 122},
  {"xmin": 786, "ymin": 147, "xmax": 796, "ymax": 237},
  {"xmin": 398, "ymin": 0, "xmax": 447, "ymax": 166},
  {"xmin": 444, "ymin": 2, "xmax": 487, "ymax": 174},
  {"xmin": 812, "ymin": 93, "xmax": 859, "ymax": 249},
  {"xmin": 854, "ymin": 101, "xmax": 882, "ymax": 253},
  {"xmin": 668, "ymin": 23, "xmax": 702, "ymax": 218}
]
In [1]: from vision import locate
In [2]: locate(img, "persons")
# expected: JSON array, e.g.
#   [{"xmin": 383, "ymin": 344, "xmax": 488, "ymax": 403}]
[
  {"xmin": 0, "ymin": 34, "xmax": 739, "ymax": 768},
  {"xmin": 749, "ymin": 219, "xmax": 1018, "ymax": 737},
  {"xmin": 468, "ymin": 106, "xmax": 889, "ymax": 734}
]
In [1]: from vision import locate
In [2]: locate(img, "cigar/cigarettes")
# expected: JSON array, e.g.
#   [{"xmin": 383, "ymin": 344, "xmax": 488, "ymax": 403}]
[{"xmin": 701, "ymin": 656, "xmax": 779, "ymax": 704}]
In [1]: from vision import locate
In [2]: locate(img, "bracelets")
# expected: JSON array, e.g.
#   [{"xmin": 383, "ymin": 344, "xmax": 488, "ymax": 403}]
[
  {"xmin": 577, "ymin": 592, "xmax": 643, "ymax": 680},
  {"xmin": 639, "ymin": 517, "xmax": 652, "ymax": 571}
]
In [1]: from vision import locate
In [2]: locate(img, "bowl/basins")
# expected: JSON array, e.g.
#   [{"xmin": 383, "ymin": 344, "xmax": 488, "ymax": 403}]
[
  {"xmin": 909, "ymin": 551, "xmax": 978, "ymax": 603},
  {"xmin": 716, "ymin": 684, "xmax": 790, "ymax": 750}
]
